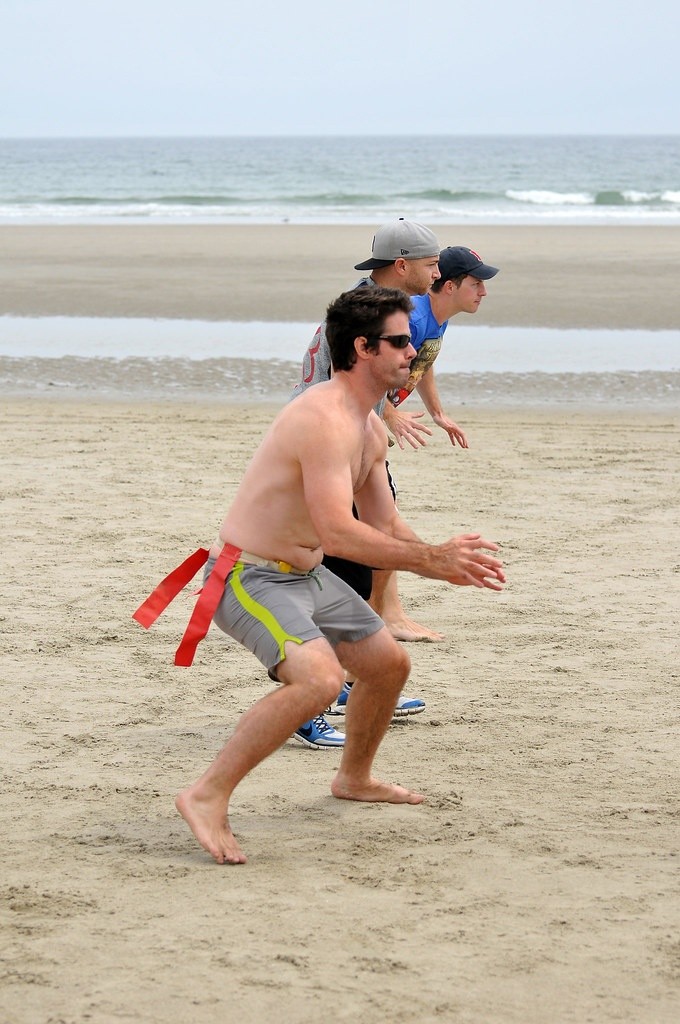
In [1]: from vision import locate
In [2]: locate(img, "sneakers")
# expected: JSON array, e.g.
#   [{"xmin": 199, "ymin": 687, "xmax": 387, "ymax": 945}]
[
  {"xmin": 335, "ymin": 680, "xmax": 425, "ymax": 717},
  {"xmin": 289, "ymin": 714, "xmax": 346, "ymax": 750}
]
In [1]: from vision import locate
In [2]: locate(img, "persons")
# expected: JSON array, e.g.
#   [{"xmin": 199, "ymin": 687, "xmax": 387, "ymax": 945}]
[
  {"xmin": 381, "ymin": 246, "xmax": 499, "ymax": 641},
  {"xmin": 287, "ymin": 218, "xmax": 444, "ymax": 753},
  {"xmin": 174, "ymin": 283, "xmax": 510, "ymax": 864}
]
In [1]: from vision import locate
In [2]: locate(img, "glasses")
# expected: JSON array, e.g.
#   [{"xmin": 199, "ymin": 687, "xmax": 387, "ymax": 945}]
[{"xmin": 366, "ymin": 333, "xmax": 412, "ymax": 349}]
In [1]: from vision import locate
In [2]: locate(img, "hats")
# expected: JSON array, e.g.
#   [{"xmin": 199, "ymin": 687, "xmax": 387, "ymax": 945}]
[
  {"xmin": 438, "ymin": 246, "xmax": 499, "ymax": 279},
  {"xmin": 354, "ymin": 218, "xmax": 440, "ymax": 270}
]
